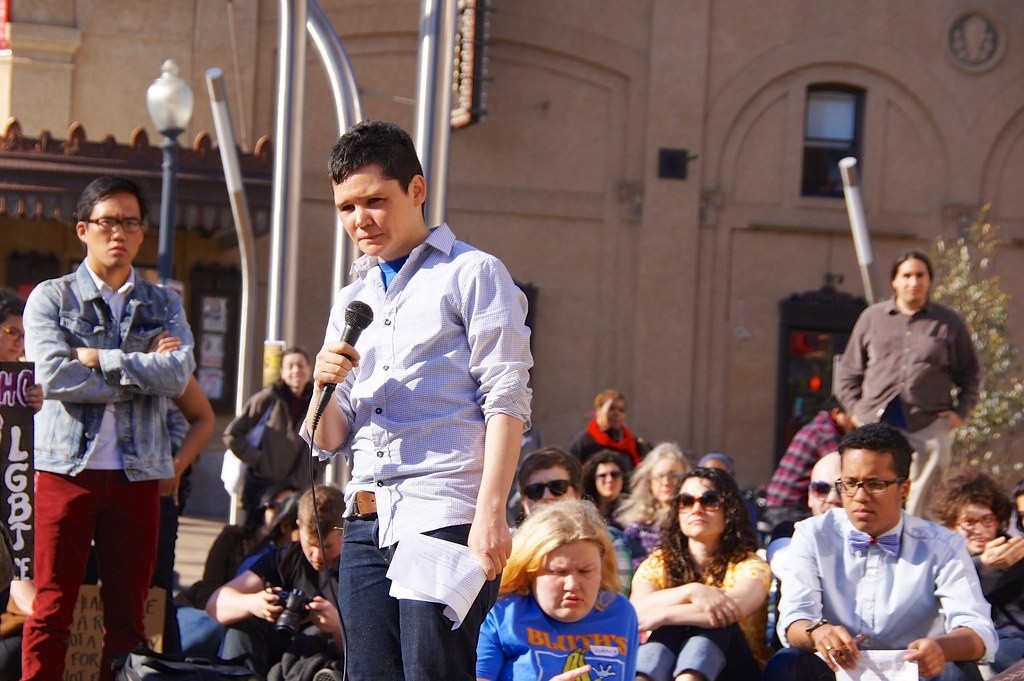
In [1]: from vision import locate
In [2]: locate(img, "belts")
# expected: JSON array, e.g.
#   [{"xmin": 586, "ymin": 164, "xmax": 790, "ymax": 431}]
[{"xmin": 348, "ymin": 490, "xmax": 379, "ymax": 517}]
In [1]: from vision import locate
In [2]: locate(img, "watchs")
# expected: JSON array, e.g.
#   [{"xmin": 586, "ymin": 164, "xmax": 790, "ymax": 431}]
[{"xmin": 804, "ymin": 618, "xmax": 830, "ymax": 647}]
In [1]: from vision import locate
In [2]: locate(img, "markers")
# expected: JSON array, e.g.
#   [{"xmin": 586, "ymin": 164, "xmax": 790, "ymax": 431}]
[{"xmin": 835, "ymin": 633, "xmax": 866, "ymax": 657}]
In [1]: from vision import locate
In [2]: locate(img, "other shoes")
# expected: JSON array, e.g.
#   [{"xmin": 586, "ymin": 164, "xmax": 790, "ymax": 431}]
[{"xmin": 314, "ymin": 668, "xmax": 343, "ymax": 681}]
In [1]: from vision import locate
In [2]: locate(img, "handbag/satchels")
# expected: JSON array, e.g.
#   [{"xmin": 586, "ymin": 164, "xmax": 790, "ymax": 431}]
[
  {"xmin": 220, "ymin": 395, "xmax": 278, "ymax": 494},
  {"xmin": 111, "ymin": 652, "xmax": 264, "ymax": 681}
]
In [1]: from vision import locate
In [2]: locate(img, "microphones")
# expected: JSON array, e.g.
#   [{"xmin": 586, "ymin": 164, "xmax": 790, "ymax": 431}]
[{"xmin": 311, "ymin": 301, "xmax": 373, "ymax": 430}]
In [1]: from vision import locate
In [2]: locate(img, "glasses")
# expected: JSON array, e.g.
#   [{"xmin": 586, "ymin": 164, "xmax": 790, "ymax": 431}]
[
  {"xmin": 0, "ymin": 326, "xmax": 26, "ymax": 343},
  {"xmin": 522, "ymin": 479, "xmax": 574, "ymax": 501},
  {"xmin": 834, "ymin": 477, "xmax": 905, "ymax": 494},
  {"xmin": 88, "ymin": 216, "xmax": 144, "ymax": 234},
  {"xmin": 807, "ymin": 481, "xmax": 841, "ymax": 499},
  {"xmin": 648, "ymin": 471, "xmax": 686, "ymax": 483},
  {"xmin": 675, "ymin": 490, "xmax": 727, "ymax": 514},
  {"xmin": 593, "ymin": 471, "xmax": 624, "ymax": 481},
  {"xmin": 955, "ymin": 514, "xmax": 1000, "ymax": 531}
]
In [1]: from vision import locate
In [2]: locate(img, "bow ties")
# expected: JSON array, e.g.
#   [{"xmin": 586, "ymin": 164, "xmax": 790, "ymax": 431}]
[{"xmin": 848, "ymin": 531, "xmax": 900, "ymax": 556}]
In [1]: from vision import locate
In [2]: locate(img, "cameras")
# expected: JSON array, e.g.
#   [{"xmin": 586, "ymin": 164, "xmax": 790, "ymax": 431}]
[{"xmin": 272, "ymin": 586, "xmax": 314, "ymax": 640}]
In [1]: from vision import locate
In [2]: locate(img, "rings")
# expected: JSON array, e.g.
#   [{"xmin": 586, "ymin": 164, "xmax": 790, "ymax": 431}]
[{"xmin": 826, "ymin": 646, "xmax": 832, "ymax": 650}]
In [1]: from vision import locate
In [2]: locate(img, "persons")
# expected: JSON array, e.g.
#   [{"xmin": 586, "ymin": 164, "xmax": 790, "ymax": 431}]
[
  {"xmin": 306, "ymin": 121, "xmax": 534, "ymax": 681},
  {"xmin": 0, "ymin": 175, "xmax": 216, "ymax": 681},
  {"xmin": 833, "ymin": 247, "xmax": 981, "ymax": 520},
  {"xmin": 472, "ymin": 390, "xmax": 1024, "ymax": 681},
  {"xmin": 193, "ymin": 349, "xmax": 345, "ymax": 681}
]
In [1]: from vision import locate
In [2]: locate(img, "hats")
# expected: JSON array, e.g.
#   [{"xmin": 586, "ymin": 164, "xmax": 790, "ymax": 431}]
[{"xmin": 699, "ymin": 452, "xmax": 736, "ymax": 477}]
